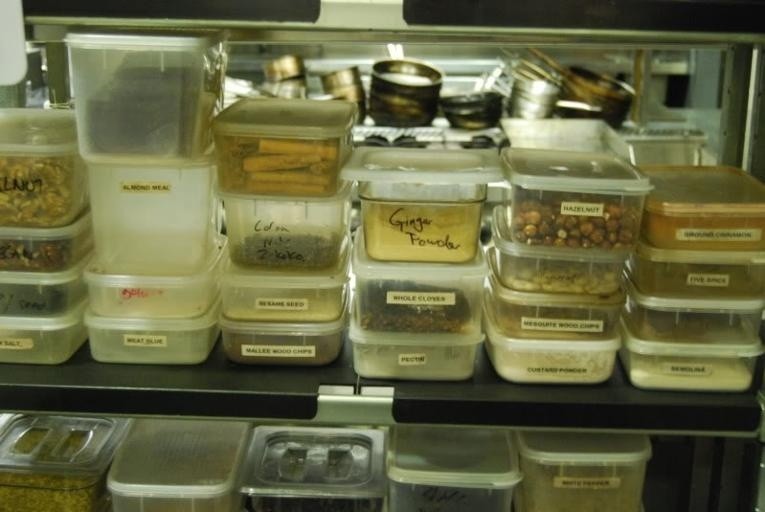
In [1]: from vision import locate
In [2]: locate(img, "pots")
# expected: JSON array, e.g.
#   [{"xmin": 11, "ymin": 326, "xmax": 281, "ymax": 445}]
[
  {"xmin": 516, "ymin": 49, "xmax": 635, "ymax": 129},
  {"xmin": 364, "ymin": 43, "xmax": 440, "ymax": 127},
  {"xmin": 443, "ymin": 66, "xmax": 504, "ymax": 130}
]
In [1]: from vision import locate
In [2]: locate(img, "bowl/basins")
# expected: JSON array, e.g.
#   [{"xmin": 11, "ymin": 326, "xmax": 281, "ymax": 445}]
[{"xmin": 263, "ymin": 55, "xmax": 305, "ymax": 97}]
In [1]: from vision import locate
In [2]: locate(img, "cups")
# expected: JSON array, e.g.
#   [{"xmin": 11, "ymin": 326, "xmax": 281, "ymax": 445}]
[
  {"xmin": 319, "ymin": 64, "xmax": 365, "ymax": 124},
  {"xmin": 509, "ymin": 80, "xmax": 559, "ymax": 121}
]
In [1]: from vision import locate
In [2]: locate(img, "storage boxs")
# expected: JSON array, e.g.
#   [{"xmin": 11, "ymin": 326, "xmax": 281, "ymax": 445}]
[
  {"xmin": 57, "ymin": 27, "xmax": 229, "ymax": 170},
  {"xmin": 80, "ymin": 152, "xmax": 222, "ymax": 277}
]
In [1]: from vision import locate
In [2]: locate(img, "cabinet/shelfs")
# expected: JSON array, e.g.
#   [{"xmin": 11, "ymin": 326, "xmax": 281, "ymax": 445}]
[{"xmin": 0, "ymin": 15, "xmax": 765, "ymax": 510}]
